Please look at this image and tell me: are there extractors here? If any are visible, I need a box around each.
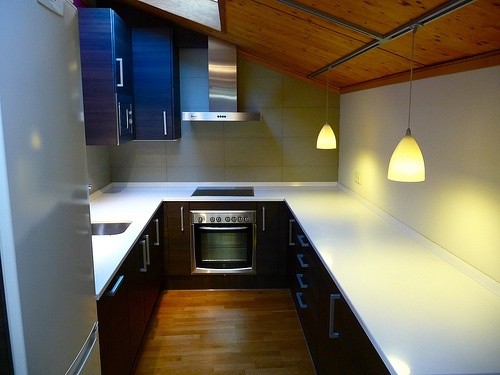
[182,35,262,121]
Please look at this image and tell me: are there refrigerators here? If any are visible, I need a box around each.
[1,0,101,375]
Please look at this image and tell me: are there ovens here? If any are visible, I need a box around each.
[189,210,257,276]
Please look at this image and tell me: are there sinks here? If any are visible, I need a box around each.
[91,224,130,236]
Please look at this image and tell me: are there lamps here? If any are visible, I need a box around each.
[317,67,338,149]
[388,25,428,183]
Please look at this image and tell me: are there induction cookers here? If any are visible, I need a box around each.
[191,187,255,196]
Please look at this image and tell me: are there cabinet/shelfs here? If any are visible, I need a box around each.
[257,202,291,278]
[131,28,184,141]
[280,215,321,363]
[126,230,162,348]
[164,202,191,278]
[97,267,134,375]
[317,269,386,375]
[79,7,134,147]
[148,210,169,293]
